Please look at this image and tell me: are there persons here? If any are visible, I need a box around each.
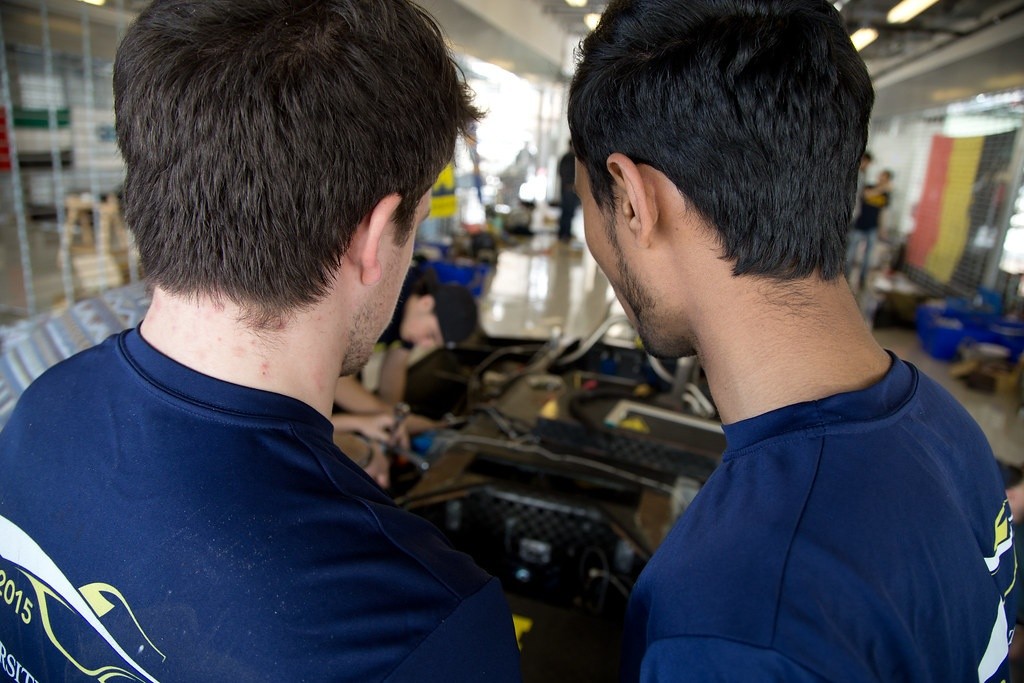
[1,1,524,683]
[845,153,895,289]
[559,141,579,239]
[570,1,1024,683]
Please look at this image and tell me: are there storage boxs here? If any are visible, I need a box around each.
[428,263,487,295]
[867,271,1024,363]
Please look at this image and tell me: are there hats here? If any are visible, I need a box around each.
[422,272,478,349]
